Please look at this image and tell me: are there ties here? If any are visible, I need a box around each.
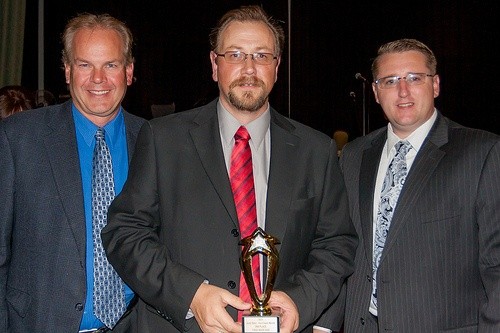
[371,140,413,308]
[230,126,262,322]
[91,127,128,330]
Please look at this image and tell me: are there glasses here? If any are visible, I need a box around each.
[217,51,277,64]
[375,73,433,88]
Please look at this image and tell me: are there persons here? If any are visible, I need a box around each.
[0,14,148,333]
[0,84,32,118]
[312,38,500,333]
[100,6,360,333]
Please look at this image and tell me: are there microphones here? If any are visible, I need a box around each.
[355,72,367,82]
